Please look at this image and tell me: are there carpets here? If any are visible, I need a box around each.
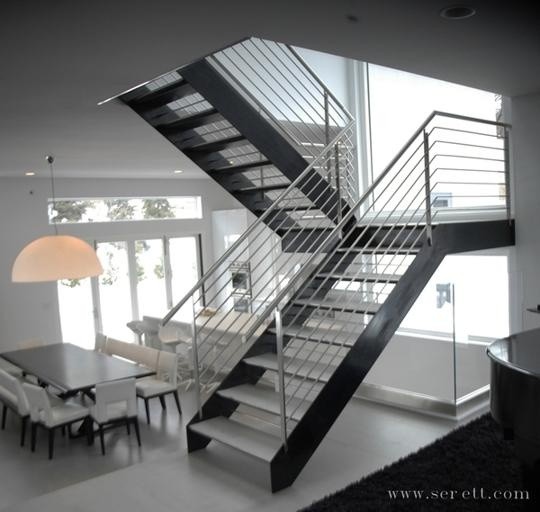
[296,410,539,512]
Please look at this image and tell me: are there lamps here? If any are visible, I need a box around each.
[11,154,104,283]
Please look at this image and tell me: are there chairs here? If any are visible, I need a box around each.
[127,320,228,392]
[1,332,183,460]
